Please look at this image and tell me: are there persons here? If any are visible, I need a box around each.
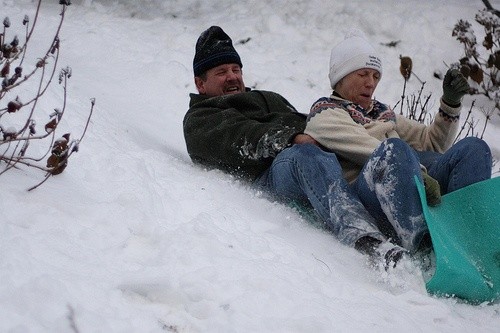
[303,37,493,206]
[182,25,410,273]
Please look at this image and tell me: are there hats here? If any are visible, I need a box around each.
[328,36,383,90]
[193,26,242,77]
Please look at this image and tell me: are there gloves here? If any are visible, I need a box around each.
[421,169,442,206]
[442,68,470,108]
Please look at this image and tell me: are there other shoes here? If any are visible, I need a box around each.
[375,238,412,274]
[420,246,437,284]
